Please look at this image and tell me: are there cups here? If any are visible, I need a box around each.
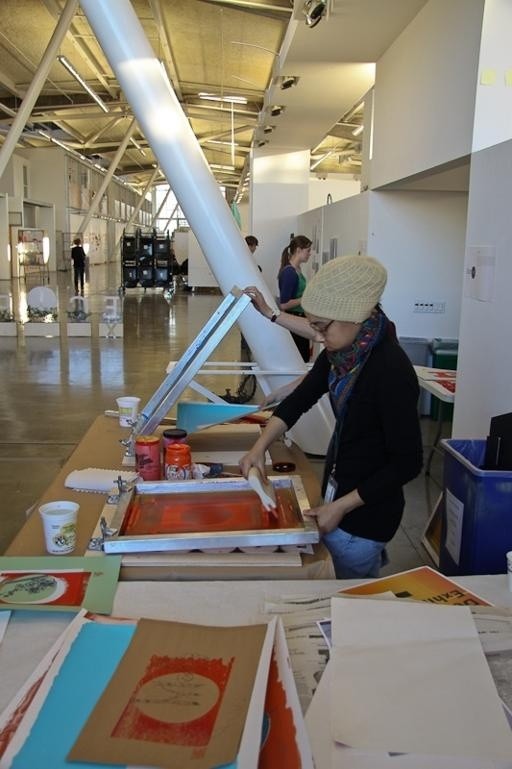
[114,395,142,427]
[37,499,82,556]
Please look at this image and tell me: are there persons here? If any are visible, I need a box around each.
[241,234,316,362]
[71,238,86,296]
[239,254,424,579]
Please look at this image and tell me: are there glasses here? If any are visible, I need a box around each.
[308,320,334,332]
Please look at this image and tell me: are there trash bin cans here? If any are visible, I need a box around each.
[439,438,512,576]
[399,337,458,422]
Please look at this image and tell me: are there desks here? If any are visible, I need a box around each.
[5,412,334,582]
[418,376,455,477]
[5,573,511,717]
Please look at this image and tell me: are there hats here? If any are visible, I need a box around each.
[300,256,388,323]
